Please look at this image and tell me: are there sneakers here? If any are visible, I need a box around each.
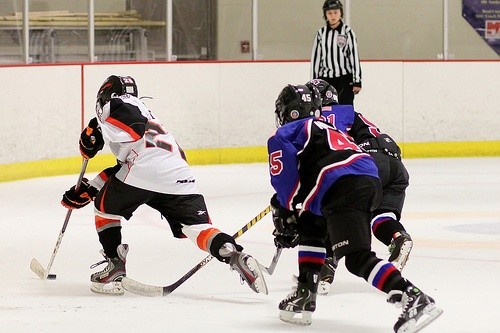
[388,230,413,273]
[278,272,319,326]
[219,242,269,295]
[386,278,443,333]
[317,253,338,294]
[90,244,129,296]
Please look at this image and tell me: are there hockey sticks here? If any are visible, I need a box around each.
[257,247,282,275]
[30,136,95,280]
[121,205,272,297]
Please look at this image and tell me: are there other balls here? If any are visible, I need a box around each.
[47,274,56,279]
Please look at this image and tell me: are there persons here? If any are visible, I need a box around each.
[60,74,268,296]
[310,0,362,105]
[260,83,446,333]
[290,78,413,291]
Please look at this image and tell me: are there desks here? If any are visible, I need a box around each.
[1,26,143,61]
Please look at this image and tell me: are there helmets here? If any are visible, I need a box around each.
[323,0,343,19]
[97,75,138,103]
[304,79,338,105]
[275,84,321,121]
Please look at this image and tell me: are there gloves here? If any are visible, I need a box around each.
[79,118,104,160]
[61,178,100,210]
[272,229,299,248]
[270,193,298,236]
